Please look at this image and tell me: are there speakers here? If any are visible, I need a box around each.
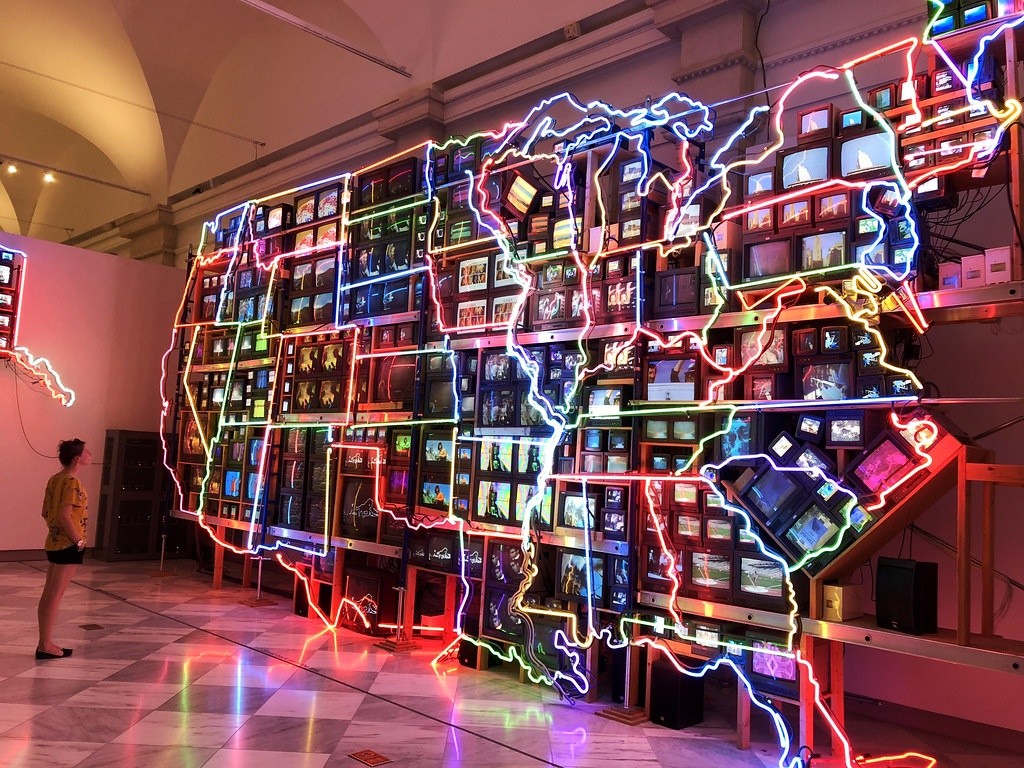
[295,577,309,618]
[650,659,704,731]
[459,639,503,669]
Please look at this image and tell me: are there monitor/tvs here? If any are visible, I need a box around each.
[175,0,1024,702]
[0,249,15,356]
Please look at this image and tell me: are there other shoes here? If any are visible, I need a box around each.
[35,647,73,659]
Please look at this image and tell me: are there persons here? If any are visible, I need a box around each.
[0,252,15,347]
[298,259,757,594]
[34,437,93,660]
